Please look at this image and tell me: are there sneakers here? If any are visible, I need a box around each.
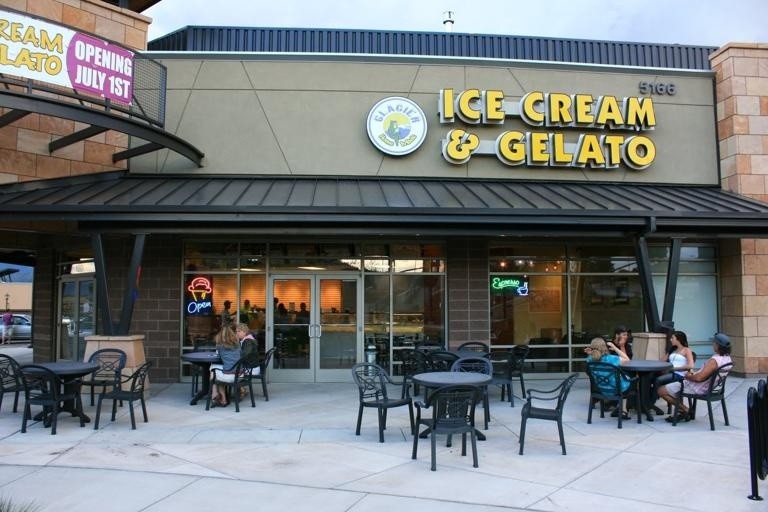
[611,408,631,419]
[665,408,690,422]
[211,394,228,408]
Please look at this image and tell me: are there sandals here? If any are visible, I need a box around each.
[240,388,248,400]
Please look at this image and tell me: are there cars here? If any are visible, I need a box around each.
[0,311,31,341]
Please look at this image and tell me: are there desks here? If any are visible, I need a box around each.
[616,360,673,421]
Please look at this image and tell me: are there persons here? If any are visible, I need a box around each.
[219,297,310,346]
[495,319,513,345]
[656,333,733,423]
[606,325,637,408]
[649,331,694,408]
[1,309,13,345]
[234,324,260,398]
[583,337,632,420]
[209,326,244,407]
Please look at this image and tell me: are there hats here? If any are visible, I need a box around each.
[709,333,731,348]
[224,301,232,305]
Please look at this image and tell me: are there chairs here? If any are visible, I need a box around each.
[95,360,154,429]
[519,373,578,455]
[0,353,32,419]
[663,351,697,413]
[586,361,641,427]
[350,333,530,471]
[77,348,127,413]
[17,365,85,435]
[673,362,734,431]
[190,331,289,412]
